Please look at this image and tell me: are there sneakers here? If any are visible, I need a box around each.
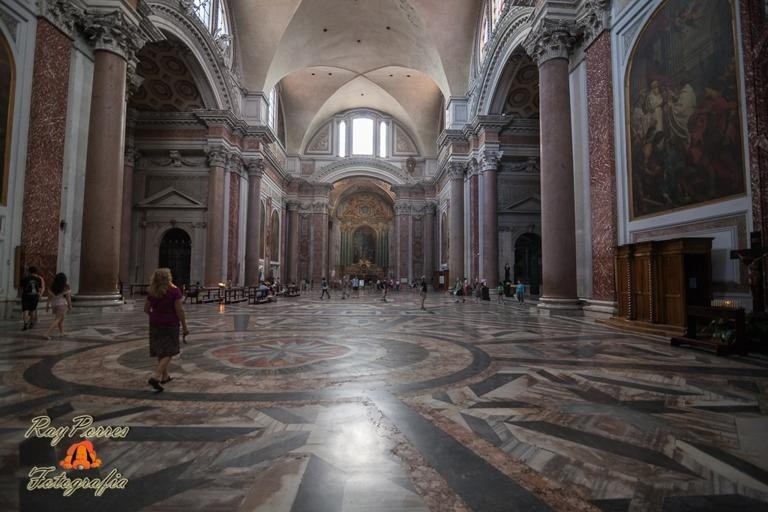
[23,322,33,330]
[42,333,51,340]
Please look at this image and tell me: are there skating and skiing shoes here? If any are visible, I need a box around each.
[60,332,68,337]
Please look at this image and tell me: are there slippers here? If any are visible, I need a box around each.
[148,378,164,392]
[160,375,175,384]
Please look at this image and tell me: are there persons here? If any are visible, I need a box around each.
[319,273,401,302]
[419,275,428,312]
[250,278,313,300]
[497,282,507,305]
[183,279,201,304]
[509,278,526,305]
[19,265,75,341]
[447,275,486,304]
[142,268,191,396]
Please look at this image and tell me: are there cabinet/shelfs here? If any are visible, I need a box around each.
[131,281,304,306]
